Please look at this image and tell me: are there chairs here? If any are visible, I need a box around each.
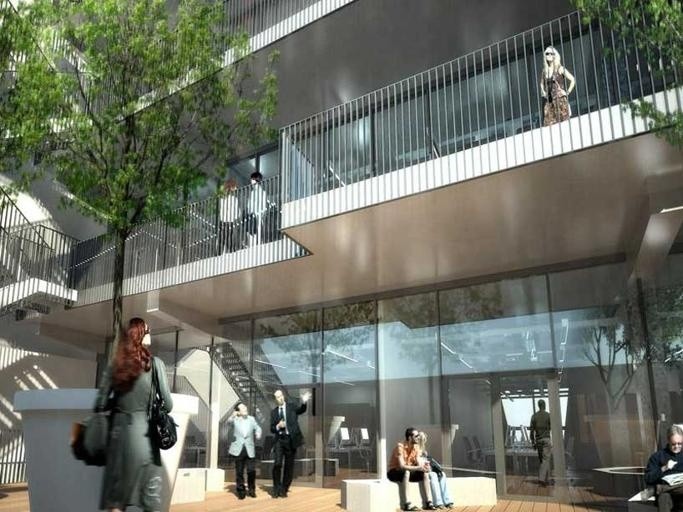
[304,432,376,472]
[463,436,574,474]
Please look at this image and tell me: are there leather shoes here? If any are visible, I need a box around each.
[249,492,256,497]
[239,493,246,499]
[273,490,287,498]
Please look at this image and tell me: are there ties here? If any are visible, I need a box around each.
[280,408,285,436]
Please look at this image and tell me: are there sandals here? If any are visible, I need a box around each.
[425,500,453,510]
[403,502,422,512]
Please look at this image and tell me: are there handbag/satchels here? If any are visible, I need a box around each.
[152,404,179,450]
[429,458,443,477]
[71,414,108,466]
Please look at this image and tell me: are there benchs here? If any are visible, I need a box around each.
[259,456,340,480]
[341,477,497,511]
[171,467,226,505]
[627,488,659,512]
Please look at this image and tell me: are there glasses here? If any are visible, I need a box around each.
[143,326,151,334]
[545,53,556,55]
[670,443,683,445]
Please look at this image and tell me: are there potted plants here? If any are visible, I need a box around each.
[0,0,281,512]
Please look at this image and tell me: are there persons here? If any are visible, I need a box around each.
[96,317,172,512]
[270,389,312,498]
[226,403,262,500]
[645,425,683,512]
[530,399,550,486]
[541,47,575,126]
[217,180,241,256]
[244,171,267,248]
[388,427,454,511]
